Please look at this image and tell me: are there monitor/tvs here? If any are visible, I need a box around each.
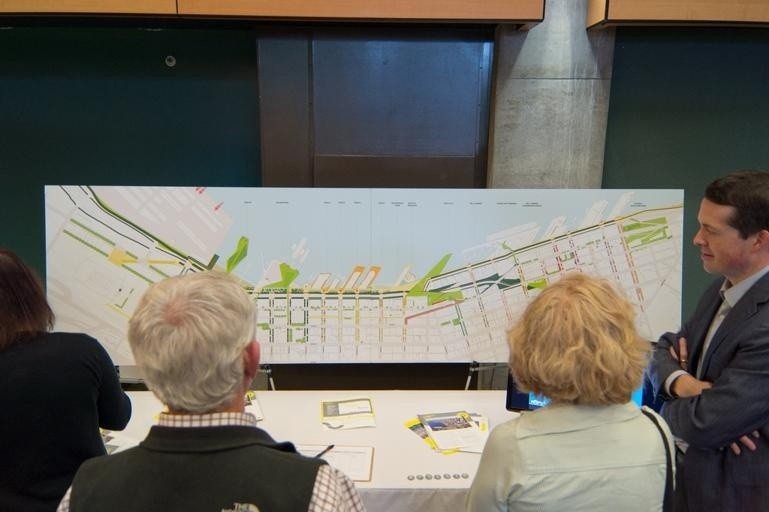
[506,341,660,412]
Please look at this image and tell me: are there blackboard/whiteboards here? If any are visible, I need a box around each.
[601,24,769,338]
[1,11,500,306]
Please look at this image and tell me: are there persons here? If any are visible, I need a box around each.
[645,168,768,511]
[0,248,132,512]
[56,268,365,511]
[466,272,676,512]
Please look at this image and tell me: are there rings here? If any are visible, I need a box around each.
[679,360,688,363]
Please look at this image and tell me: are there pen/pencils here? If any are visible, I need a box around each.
[312,443,335,459]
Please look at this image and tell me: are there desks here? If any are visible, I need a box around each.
[58,390,521,511]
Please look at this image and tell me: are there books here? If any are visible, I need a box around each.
[321,399,376,430]
[242,390,262,421]
[290,444,375,482]
[401,409,489,455]
